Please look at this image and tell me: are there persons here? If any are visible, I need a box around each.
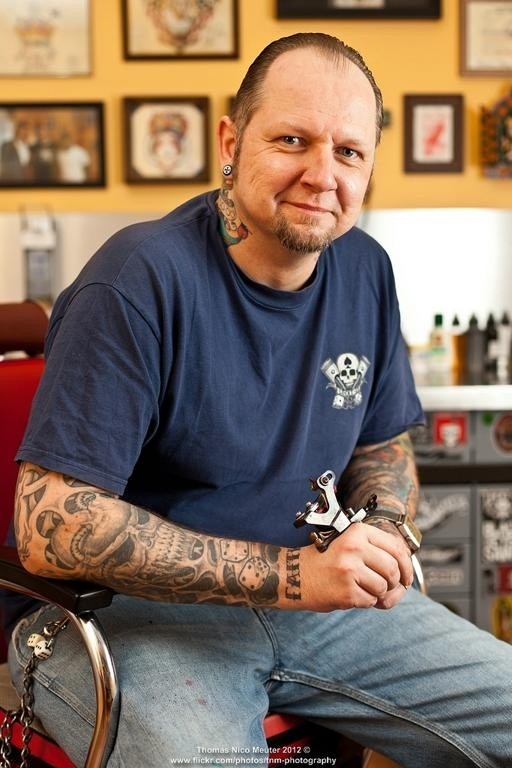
[11,30,511,768]
[47,129,94,182]
[2,122,39,182]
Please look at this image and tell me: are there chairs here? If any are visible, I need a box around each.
[0,297,324,766]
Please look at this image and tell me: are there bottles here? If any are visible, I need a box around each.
[428,310,512,386]
[19,197,66,318]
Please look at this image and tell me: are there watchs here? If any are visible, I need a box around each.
[363,506,424,554]
